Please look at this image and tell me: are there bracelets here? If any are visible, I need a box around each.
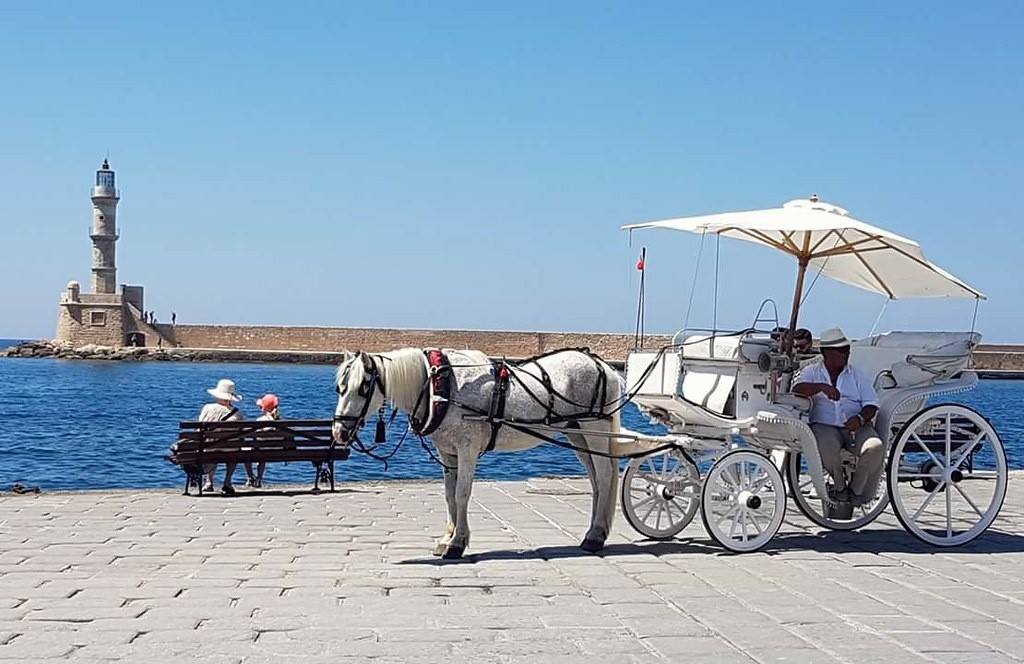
[856,413,864,427]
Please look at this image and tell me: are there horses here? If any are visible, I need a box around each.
[332,348,623,560]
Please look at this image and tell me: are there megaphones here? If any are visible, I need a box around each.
[757,352,791,374]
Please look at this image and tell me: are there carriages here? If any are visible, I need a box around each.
[333,194,1010,560]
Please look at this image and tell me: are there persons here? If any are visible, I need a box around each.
[242,394,280,487]
[776,329,820,393]
[792,328,884,507]
[196,379,243,494]
[771,327,795,358]
[132,311,176,351]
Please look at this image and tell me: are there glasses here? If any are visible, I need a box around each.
[827,345,850,354]
[794,341,809,350]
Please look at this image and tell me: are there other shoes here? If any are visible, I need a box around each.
[222,483,235,494]
[202,482,214,492]
[254,478,261,487]
[829,481,848,501]
[246,479,253,486]
[848,486,863,507]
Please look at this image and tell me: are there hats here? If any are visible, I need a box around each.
[257,394,279,412]
[207,379,242,402]
[812,328,852,347]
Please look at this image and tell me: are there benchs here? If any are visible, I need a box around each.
[164,420,351,497]
[795,330,983,423]
[671,298,779,374]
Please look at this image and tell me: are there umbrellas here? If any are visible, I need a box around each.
[620,192,986,332]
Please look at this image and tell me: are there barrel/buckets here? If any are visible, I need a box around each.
[821,475,854,519]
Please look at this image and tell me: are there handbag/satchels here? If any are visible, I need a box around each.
[284,435,296,450]
[170,438,197,455]
